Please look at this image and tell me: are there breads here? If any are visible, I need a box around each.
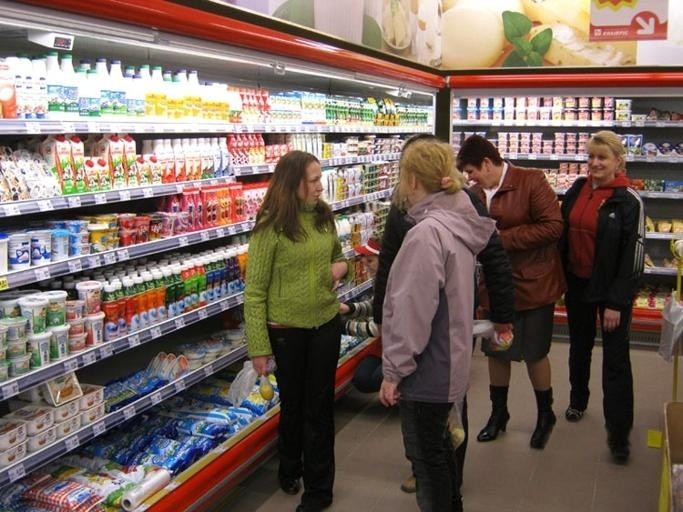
[441,0,638,70]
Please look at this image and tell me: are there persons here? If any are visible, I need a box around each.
[455,134,569,449]
[371,135,498,511]
[373,135,515,493]
[242,149,356,512]
[560,130,645,467]
[339,236,383,393]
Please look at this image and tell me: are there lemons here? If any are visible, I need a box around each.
[260,379,274,400]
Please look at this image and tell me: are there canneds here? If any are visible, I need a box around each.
[564,96,615,121]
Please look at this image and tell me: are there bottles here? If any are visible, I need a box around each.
[0,51,270,122]
[37,242,251,344]
[154,180,271,236]
[140,132,266,185]
[334,213,352,251]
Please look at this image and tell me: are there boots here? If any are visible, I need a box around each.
[478,385,509,441]
[531,387,556,448]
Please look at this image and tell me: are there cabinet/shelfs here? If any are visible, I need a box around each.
[449,64,683,355]
[0,0,449,511]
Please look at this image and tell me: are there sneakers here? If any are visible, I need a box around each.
[281,477,299,494]
[566,405,584,421]
[402,476,416,492]
